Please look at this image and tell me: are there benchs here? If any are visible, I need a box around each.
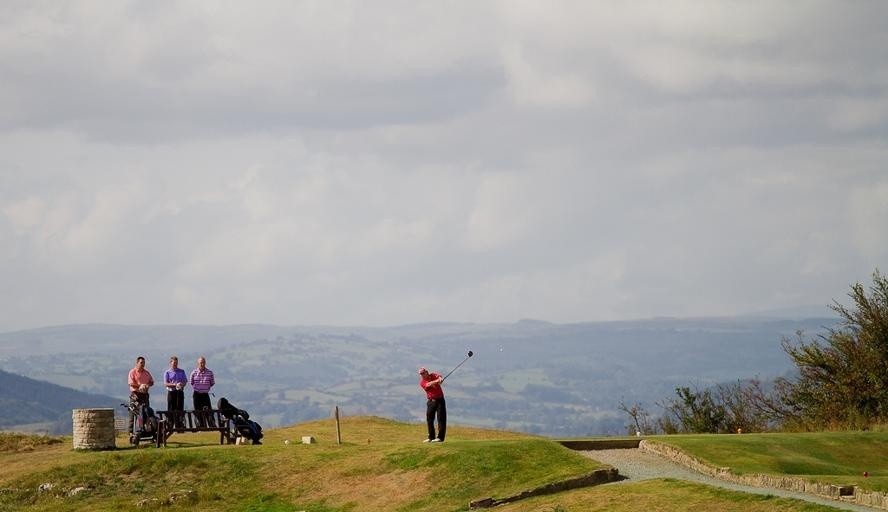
[155,409,233,448]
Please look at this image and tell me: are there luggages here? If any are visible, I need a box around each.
[217,397,264,444]
[129,401,165,447]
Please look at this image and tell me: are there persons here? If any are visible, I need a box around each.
[163,357,188,433]
[417,366,448,443]
[126,356,156,437]
[190,355,218,428]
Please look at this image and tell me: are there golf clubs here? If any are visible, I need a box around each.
[443,351,473,379]
[120,402,139,415]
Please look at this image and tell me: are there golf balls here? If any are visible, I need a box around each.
[284,440,289,444]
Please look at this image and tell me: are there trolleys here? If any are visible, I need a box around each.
[125,408,163,447]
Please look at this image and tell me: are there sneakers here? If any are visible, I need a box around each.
[423,439,442,443]
